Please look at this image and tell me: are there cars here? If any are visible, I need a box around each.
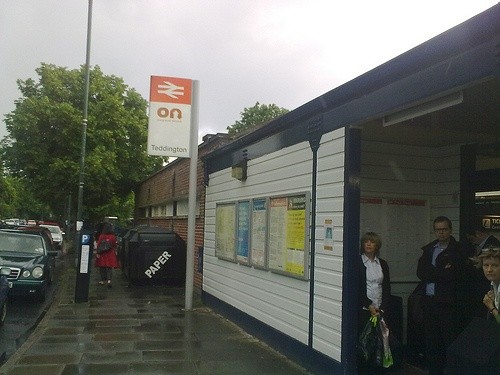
[0,218,66,324]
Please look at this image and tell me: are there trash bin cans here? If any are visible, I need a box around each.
[121,226,187,285]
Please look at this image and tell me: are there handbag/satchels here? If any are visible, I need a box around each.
[98,243,110,253]
[360,317,393,368]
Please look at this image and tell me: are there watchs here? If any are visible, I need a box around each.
[489,307,497,314]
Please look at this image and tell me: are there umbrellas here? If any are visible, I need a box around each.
[444,317,500,375]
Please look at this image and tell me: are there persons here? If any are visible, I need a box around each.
[358,231,391,375]
[469,246,499,324]
[95,223,119,289]
[417,215,468,364]
[462,221,500,300]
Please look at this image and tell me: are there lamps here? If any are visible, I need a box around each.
[232,159,247,181]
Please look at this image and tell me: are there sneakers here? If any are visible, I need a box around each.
[108,280,110,283]
[99,281,103,284]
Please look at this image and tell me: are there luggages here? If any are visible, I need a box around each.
[386,294,402,367]
[408,280,428,368]
